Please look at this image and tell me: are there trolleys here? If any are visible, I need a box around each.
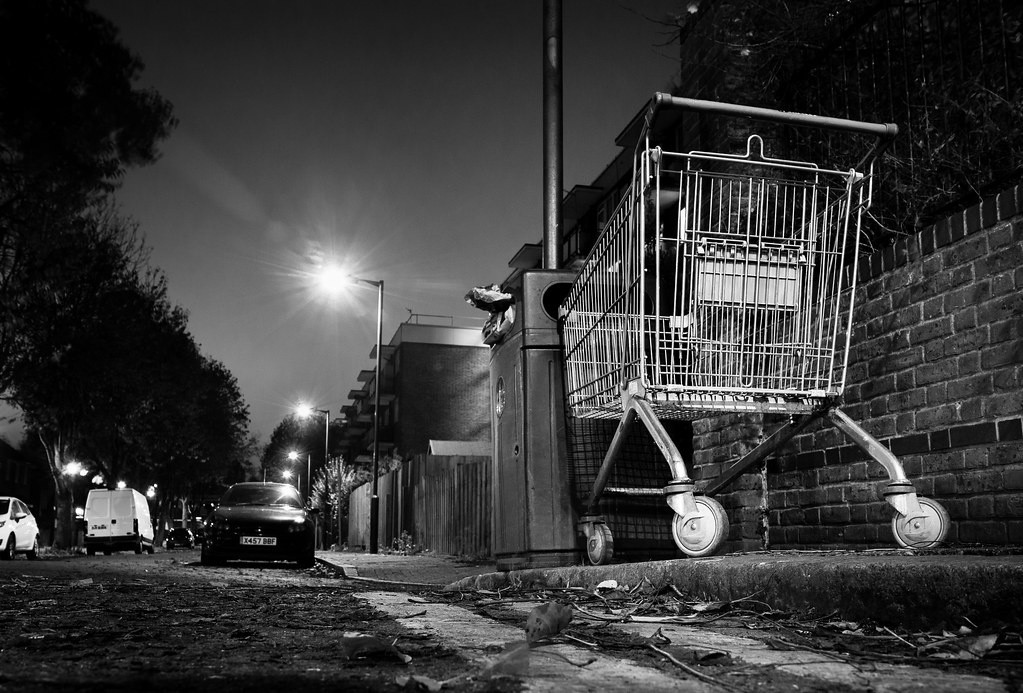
[558,83,953,563]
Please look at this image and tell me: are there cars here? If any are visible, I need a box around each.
[166,527,195,550]
[0,496,42,560]
[201,482,317,569]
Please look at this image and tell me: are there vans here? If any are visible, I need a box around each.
[81,485,156,554]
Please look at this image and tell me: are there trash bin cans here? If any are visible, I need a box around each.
[464,267,696,570]
